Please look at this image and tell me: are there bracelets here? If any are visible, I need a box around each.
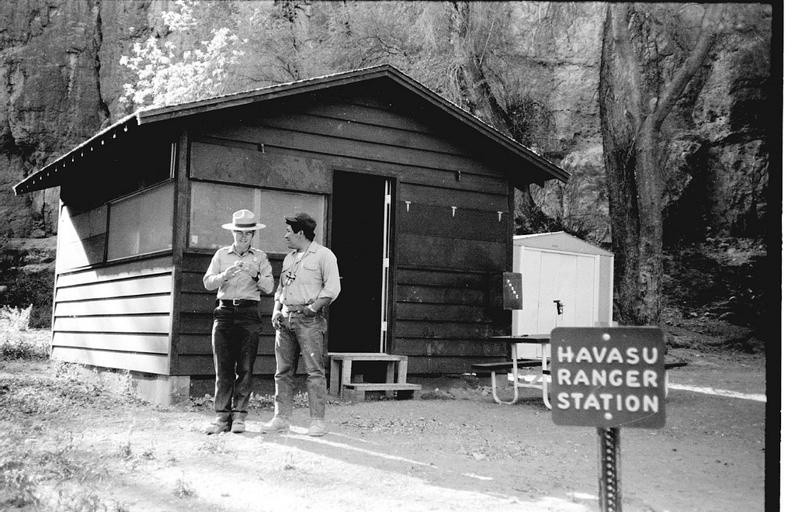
[307,304,317,314]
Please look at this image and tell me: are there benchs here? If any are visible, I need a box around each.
[472,358,550,403]
[542,360,687,399]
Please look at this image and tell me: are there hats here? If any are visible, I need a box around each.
[221,210,266,231]
[285,212,317,232]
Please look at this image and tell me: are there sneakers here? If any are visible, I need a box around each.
[204,419,245,434]
[309,419,327,436]
[260,416,291,433]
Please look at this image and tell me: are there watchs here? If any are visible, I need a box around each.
[251,272,262,282]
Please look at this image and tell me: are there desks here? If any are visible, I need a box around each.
[491,335,554,410]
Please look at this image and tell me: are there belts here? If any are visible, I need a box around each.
[219,299,257,307]
[282,305,304,312]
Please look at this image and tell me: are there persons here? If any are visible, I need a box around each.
[258,211,342,437]
[201,210,276,434]
